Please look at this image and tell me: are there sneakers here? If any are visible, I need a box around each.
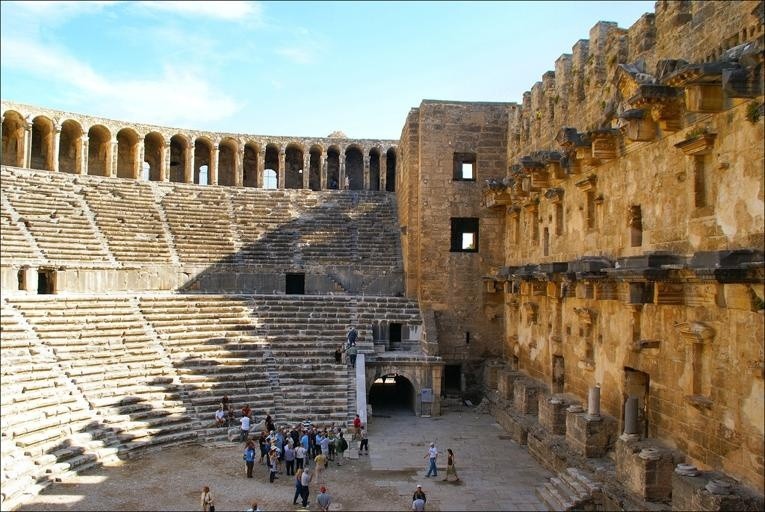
[424,474,438,478]
[292,501,310,509]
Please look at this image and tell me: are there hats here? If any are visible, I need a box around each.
[417,484,422,488]
[319,486,327,492]
[429,442,435,446]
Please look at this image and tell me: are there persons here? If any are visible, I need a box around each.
[442,449,462,482]
[247,503,261,512]
[215,392,235,428]
[423,442,439,477]
[201,486,214,512]
[328,175,349,190]
[293,468,313,507]
[316,487,331,512]
[334,328,357,368]
[240,403,368,483]
[411,484,426,512]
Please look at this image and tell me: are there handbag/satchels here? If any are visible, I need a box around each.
[210,506,215,512]
